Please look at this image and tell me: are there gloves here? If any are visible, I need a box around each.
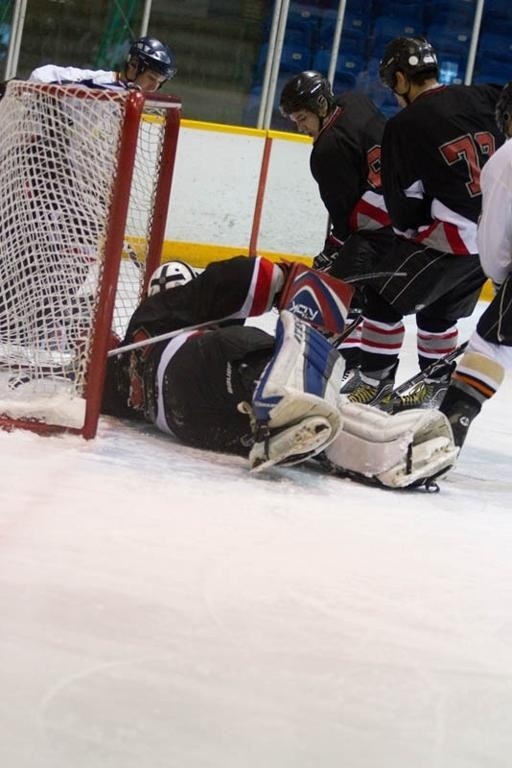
[311,237,341,271]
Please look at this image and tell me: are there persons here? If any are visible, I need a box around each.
[437,78,512,481]
[346,32,505,414]
[1,36,179,355]
[84,257,456,490]
[277,70,390,397]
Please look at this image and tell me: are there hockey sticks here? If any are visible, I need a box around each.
[8,271,409,390]
[367,339,470,415]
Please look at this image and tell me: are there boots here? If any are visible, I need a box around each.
[344,361,397,407]
[338,364,360,394]
[393,361,458,414]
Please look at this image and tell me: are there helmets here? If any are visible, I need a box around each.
[146,260,196,296]
[278,70,331,119]
[126,37,178,84]
[377,35,439,86]
